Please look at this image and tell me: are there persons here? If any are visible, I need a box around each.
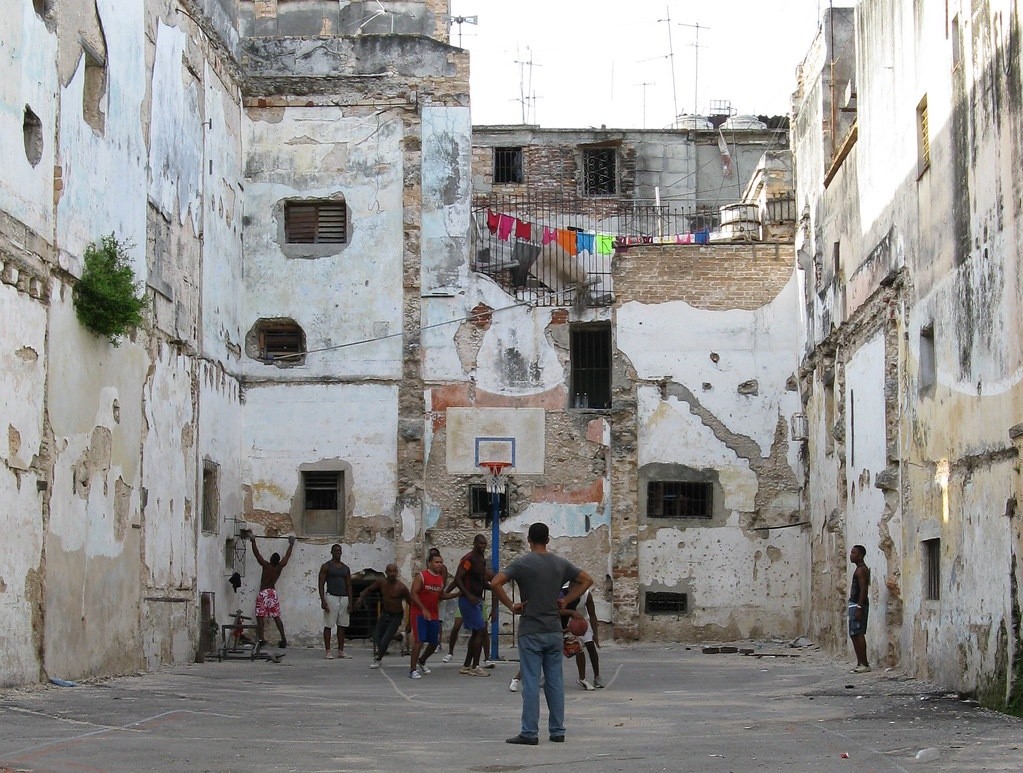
[491,522,594,746]
[246,533,295,648]
[508,579,581,692]
[401,548,447,656]
[318,543,354,660]
[354,562,411,669]
[561,580,605,688]
[408,552,463,682]
[454,533,490,677]
[846,544,872,674]
[444,577,494,668]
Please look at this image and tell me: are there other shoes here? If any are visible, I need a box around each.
[254,639,266,648]
[550,735,565,742]
[505,735,538,745]
[279,640,286,647]
[850,665,858,674]
[854,663,871,673]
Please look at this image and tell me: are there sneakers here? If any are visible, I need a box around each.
[337,650,353,658]
[369,659,382,668]
[409,670,422,679]
[416,659,430,673]
[484,660,496,668]
[593,677,605,687]
[460,665,471,673]
[576,677,596,690]
[469,665,490,677]
[510,678,519,692]
[443,654,453,663]
[325,650,333,659]
[399,631,408,648]
[540,677,546,687]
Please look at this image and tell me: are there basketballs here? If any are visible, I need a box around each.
[570,618,588,636]
